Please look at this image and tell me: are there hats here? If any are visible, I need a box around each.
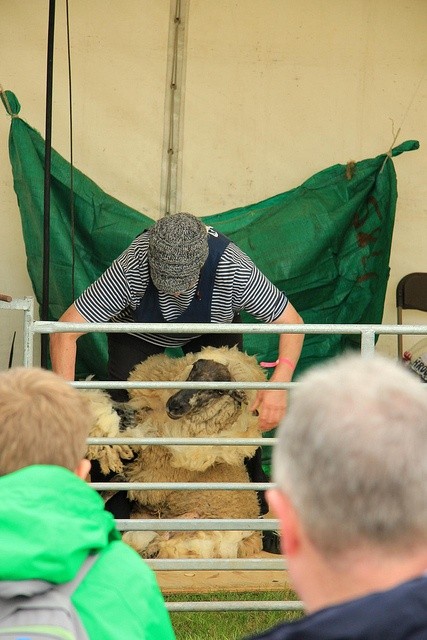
[148,212,209,292]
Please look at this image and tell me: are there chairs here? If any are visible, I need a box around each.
[395,272,427,383]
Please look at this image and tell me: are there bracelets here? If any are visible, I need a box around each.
[259,354,298,369]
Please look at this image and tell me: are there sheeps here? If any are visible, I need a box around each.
[100,345,271,559]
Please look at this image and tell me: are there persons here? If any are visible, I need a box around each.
[1,367,175,640]
[248,350,425,640]
[49,212,305,483]
